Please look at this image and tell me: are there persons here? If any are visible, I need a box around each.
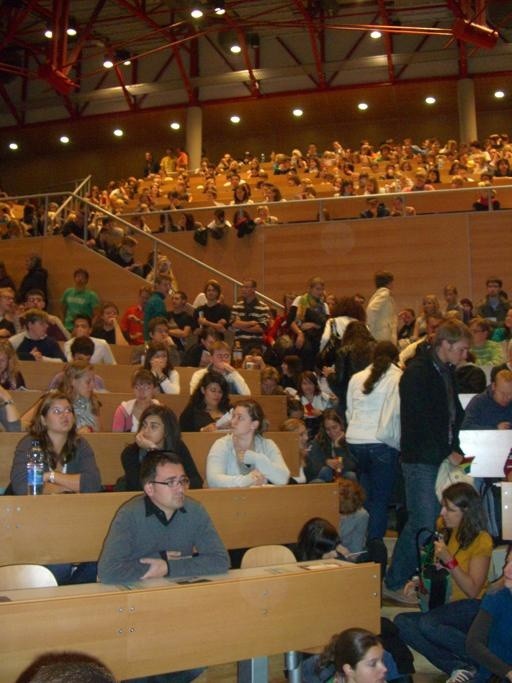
[1,185,159,277]
[109,133,512,231]
[1,253,511,683]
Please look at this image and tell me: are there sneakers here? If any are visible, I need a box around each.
[447,667,479,683]
[381,580,420,604]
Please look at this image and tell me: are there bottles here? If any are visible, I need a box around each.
[233,340,246,369]
[260,153,265,162]
[199,311,204,327]
[26,440,43,495]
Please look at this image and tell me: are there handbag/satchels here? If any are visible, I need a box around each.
[316,336,342,374]
[416,537,450,612]
[435,459,473,502]
[376,379,400,453]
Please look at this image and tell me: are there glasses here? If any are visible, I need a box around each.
[149,478,190,487]
